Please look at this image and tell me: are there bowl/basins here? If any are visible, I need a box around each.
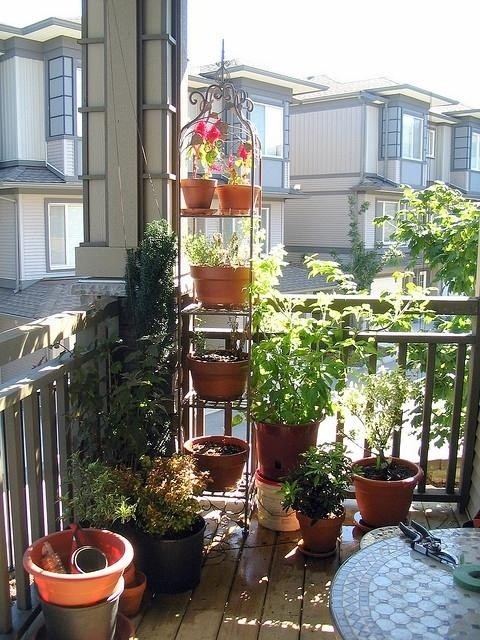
[23,529,136,607]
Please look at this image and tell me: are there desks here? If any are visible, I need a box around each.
[329,526,480,640]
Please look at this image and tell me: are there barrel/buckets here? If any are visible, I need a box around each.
[253,479,301,533]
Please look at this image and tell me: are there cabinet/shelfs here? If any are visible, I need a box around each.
[177,61,262,537]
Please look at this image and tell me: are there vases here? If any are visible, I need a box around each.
[116,613,139,640]
[216,184,262,214]
[180,177,217,209]
[192,267,253,308]
[118,571,147,616]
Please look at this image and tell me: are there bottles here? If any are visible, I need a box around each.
[41,541,67,575]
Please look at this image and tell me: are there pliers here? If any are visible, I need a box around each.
[399,520,458,568]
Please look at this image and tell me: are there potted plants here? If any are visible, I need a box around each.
[334,365,425,525]
[113,454,214,591]
[186,315,253,401]
[231,253,440,481]
[273,442,364,552]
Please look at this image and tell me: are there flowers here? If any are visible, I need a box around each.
[181,230,249,266]
[182,117,222,179]
[224,143,251,185]
